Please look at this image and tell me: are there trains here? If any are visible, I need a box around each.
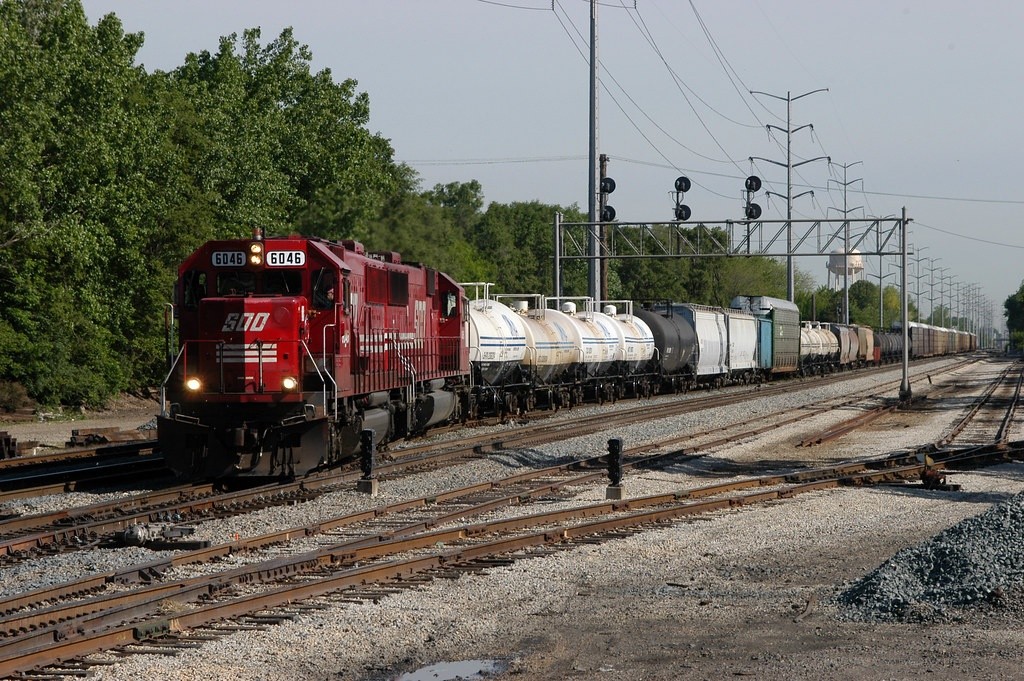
[155,223,982,499]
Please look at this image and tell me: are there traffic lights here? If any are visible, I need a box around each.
[606,437,624,482]
[359,428,377,476]
